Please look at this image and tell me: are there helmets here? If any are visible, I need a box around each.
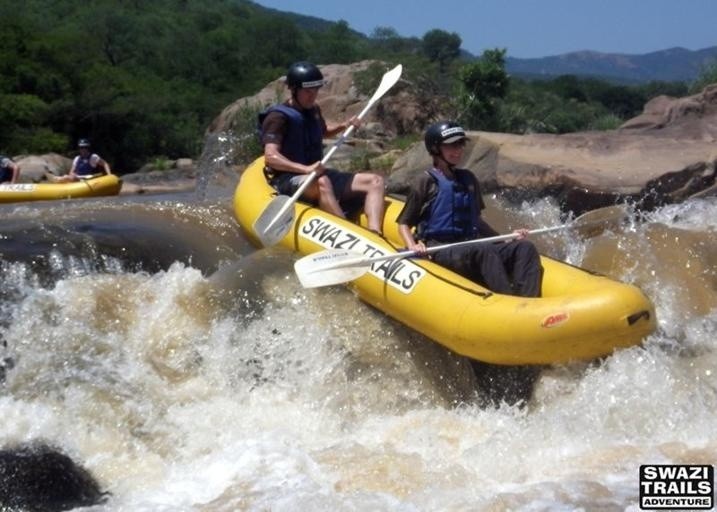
[78,139,90,147]
[425,121,471,156]
[288,63,323,89]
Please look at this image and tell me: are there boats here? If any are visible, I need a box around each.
[0,173,124,204]
[231,154,659,367]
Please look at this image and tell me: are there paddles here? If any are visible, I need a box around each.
[44,171,103,184]
[253,62,404,245]
[293,207,621,289]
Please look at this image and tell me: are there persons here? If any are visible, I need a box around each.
[256,60,386,234]
[396,122,543,297]
[70,139,111,178]
[0,156,19,185]
